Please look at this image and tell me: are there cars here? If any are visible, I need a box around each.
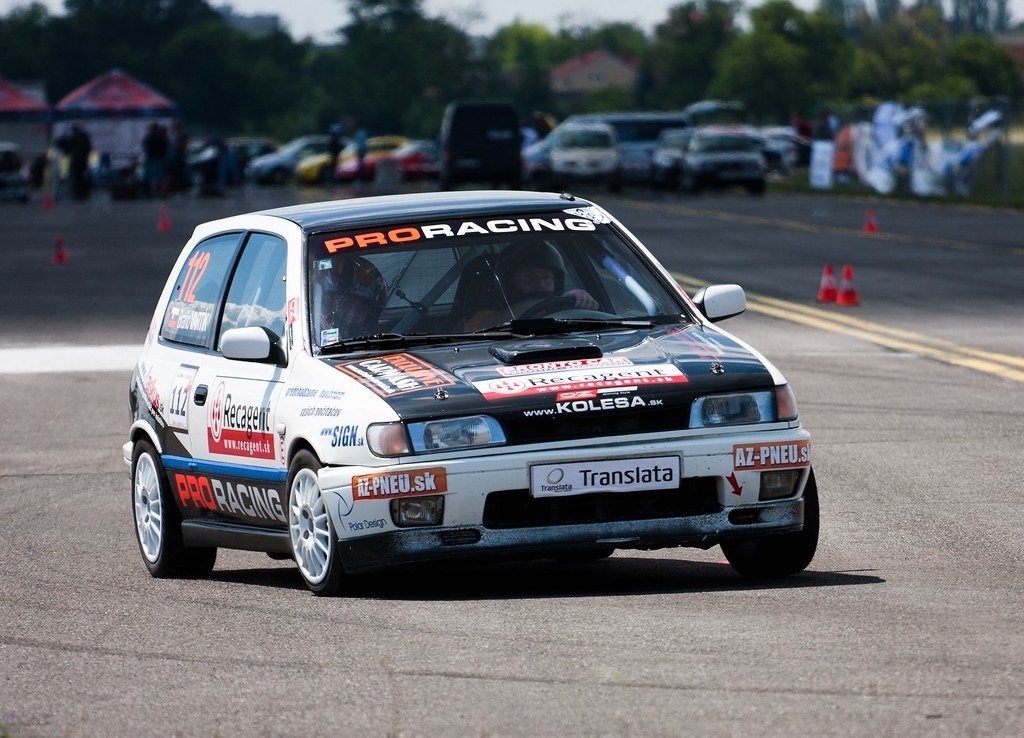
[182,99,801,198]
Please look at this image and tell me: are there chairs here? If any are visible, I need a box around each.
[446,253,497,333]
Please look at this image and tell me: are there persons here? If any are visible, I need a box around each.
[464,239,599,332]
[314,257,387,340]
[61,107,929,200]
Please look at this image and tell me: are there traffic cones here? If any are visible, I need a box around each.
[862,208,878,233]
[155,206,171,230]
[816,263,838,303]
[42,190,53,210]
[52,233,69,264]
[837,263,860,308]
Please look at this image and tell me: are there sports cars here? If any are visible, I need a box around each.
[119,188,821,597]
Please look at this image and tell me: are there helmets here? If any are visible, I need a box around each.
[498,239,566,303]
[314,255,387,339]
